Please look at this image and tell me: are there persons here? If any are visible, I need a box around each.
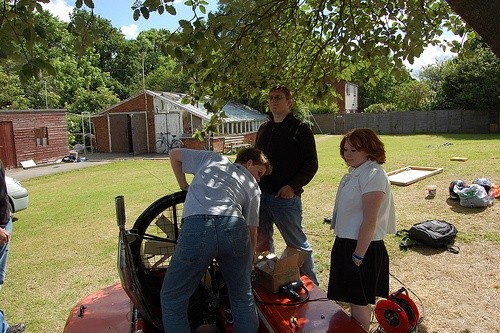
[0,157,25,333]
[253,86,321,287]
[160,146,272,333]
[327,127,396,333]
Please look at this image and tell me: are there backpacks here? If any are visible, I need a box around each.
[396,218,458,253]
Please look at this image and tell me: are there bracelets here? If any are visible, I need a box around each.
[352,252,364,261]
[180,184,189,190]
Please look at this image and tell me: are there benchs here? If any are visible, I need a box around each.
[5,175,29,212]
[223,135,252,155]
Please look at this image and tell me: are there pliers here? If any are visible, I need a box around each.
[290,313,298,329]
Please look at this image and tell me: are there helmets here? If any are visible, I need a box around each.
[448,180,464,200]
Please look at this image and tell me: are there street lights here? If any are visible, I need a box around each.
[142,51,149,152]
[40,80,48,109]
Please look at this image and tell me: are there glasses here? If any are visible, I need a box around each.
[268,95,283,100]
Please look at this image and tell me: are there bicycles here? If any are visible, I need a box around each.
[153,131,184,155]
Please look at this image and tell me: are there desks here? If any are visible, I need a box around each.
[253,273,370,333]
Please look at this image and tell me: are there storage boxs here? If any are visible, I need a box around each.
[254,247,308,294]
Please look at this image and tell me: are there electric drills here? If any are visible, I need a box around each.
[280,281,304,299]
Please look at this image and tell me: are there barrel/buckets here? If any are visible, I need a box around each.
[428,188,436,196]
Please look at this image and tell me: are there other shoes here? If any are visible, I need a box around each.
[6,323,25,333]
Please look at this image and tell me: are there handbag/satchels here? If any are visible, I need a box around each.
[453,183,489,208]
[473,178,492,193]
